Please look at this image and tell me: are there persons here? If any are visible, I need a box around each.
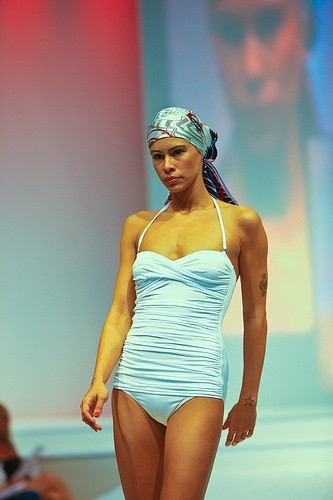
[0,403,74,500]
[198,0,333,416]
[78,107,269,500]
